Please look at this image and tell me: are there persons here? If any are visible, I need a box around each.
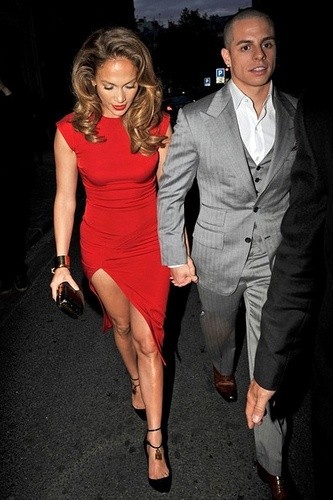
[155,10,298,500]
[245,79,333,500]
[49,26,195,492]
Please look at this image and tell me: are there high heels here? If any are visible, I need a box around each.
[143,427,171,493]
[130,377,147,421]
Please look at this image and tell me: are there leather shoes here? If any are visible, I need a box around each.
[213,365,238,402]
[267,473,289,500]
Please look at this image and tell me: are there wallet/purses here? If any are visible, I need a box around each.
[56,266,85,320]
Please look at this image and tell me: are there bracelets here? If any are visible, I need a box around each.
[54,254,71,268]
